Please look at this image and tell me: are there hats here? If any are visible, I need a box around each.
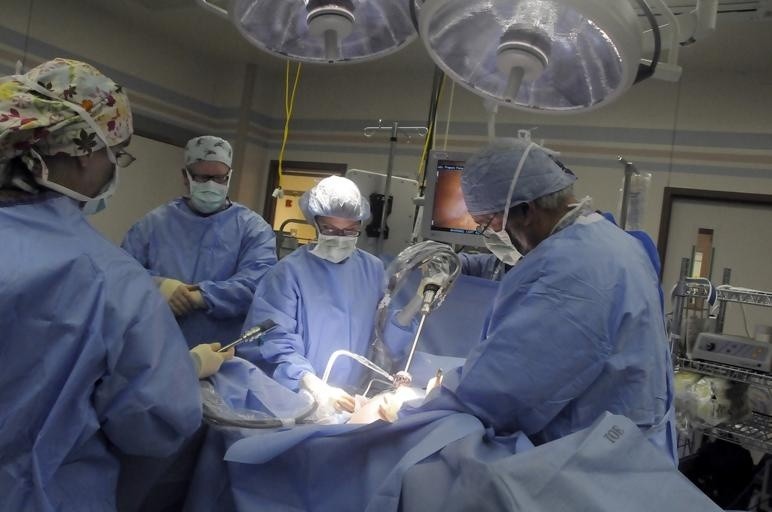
[0,57,135,165]
[183,135,234,169]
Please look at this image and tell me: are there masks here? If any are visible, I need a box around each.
[183,168,233,214]
[1,59,120,216]
[480,140,560,267]
[307,221,359,265]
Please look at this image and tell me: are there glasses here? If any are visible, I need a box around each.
[115,148,135,169]
[472,211,503,241]
[190,174,229,184]
[315,221,362,238]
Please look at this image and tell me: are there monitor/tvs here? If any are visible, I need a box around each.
[420,150,487,248]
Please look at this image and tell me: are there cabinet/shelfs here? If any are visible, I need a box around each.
[656,256,772,510]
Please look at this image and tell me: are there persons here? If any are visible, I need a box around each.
[237,173,393,412]
[379,136,681,473]
[457,154,664,316]
[0,56,206,512]
[120,134,281,512]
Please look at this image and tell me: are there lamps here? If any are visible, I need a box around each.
[197,0,726,115]
